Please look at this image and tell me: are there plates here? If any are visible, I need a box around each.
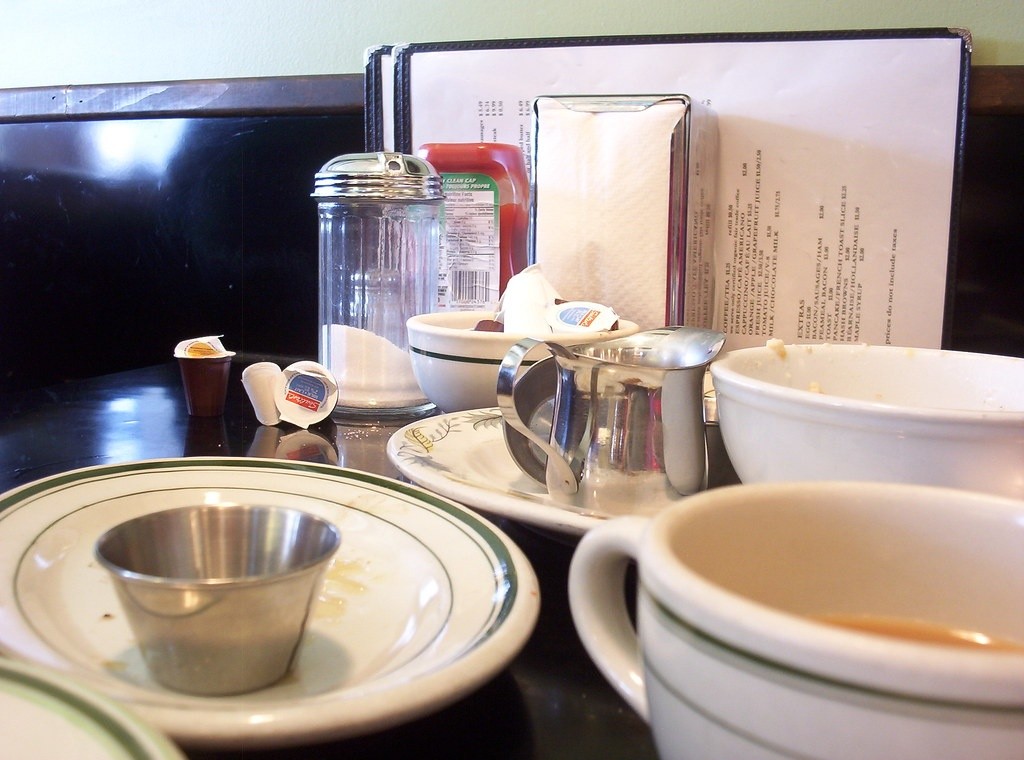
[0,457,540,747]
[0,657,188,760]
[386,406,648,535]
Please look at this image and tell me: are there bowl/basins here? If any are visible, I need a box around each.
[710,345,1024,502]
[407,311,640,413]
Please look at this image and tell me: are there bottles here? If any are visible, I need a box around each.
[310,151,443,415]
[418,143,530,309]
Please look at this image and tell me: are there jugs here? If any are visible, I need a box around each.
[498,326,726,519]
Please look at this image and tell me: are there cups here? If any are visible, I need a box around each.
[567,481,1024,760]
[96,503,339,695]
[173,351,236,416]
[702,390,742,490]
